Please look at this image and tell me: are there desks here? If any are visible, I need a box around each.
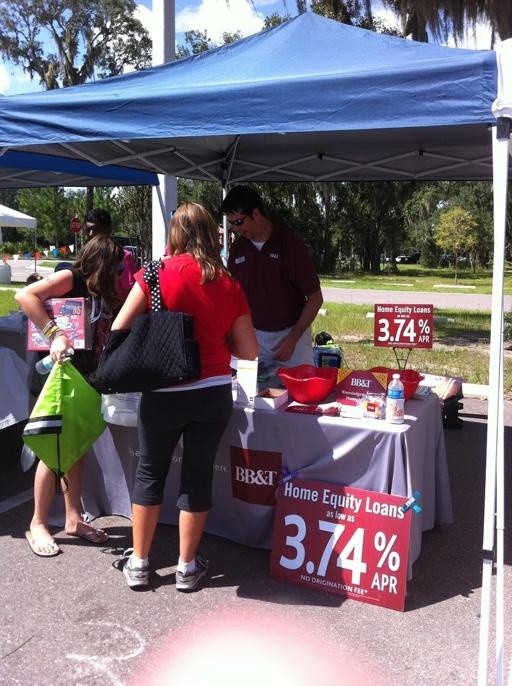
[82,384,452,594]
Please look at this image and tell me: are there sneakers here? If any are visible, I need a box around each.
[175,551,209,590]
[122,556,150,587]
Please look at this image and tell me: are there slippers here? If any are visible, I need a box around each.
[25,530,59,557]
[67,528,109,544]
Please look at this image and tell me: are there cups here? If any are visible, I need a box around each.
[386,398,405,424]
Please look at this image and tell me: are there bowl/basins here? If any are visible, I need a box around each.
[278,364,337,404]
[370,367,422,400]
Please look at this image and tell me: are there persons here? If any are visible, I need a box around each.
[111,199,260,592]
[82,208,138,317]
[221,186,325,384]
[216,224,230,268]
[15,232,125,556]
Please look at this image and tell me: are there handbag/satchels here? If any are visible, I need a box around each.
[22,356,108,476]
[94,311,201,394]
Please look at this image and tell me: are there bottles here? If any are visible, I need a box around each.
[34,347,74,375]
[388,373,404,398]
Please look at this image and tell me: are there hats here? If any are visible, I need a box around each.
[86,209,111,224]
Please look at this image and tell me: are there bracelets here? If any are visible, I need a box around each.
[42,320,64,342]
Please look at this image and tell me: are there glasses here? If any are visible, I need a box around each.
[227,214,247,226]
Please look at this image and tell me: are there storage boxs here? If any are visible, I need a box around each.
[26,299,96,351]
